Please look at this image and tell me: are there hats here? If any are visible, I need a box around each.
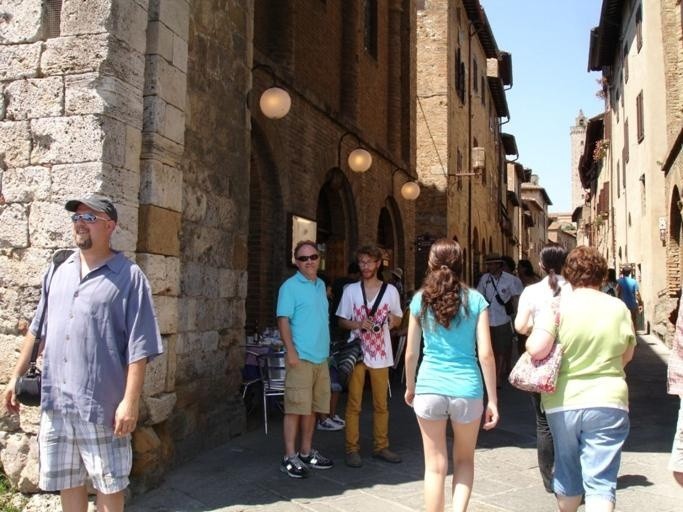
[64,193,117,225]
[483,255,504,264]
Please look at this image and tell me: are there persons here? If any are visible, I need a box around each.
[527,247,637,510]
[405,239,500,512]
[667,290,683,491]
[514,242,570,493]
[334,264,404,338]
[277,242,333,479]
[336,246,403,468]
[4,193,164,512]
[471,254,544,392]
[316,272,345,434]
[599,263,643,332]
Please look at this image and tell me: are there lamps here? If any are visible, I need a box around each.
[337,132,373,173]
[251,63,291,120]
[392,168,420,200]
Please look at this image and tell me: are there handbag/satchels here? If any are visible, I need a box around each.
[505,297,514,315]
[507,343,566,393]
[336,337,361,376]
[14,368,41,406]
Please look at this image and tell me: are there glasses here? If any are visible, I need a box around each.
[296,255,319,261]
[69,213,108,224]
[359,259,373,266]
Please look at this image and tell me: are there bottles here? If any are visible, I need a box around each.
[263,328,270,338]
[254,320,259,343]
[272,330,279,339]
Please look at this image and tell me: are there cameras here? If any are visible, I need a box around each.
[362,320,381,335]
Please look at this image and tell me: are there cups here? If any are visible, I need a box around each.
[247,337,253,343]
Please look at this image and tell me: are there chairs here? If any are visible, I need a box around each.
[240,349,283,414]
[256,351,288,440]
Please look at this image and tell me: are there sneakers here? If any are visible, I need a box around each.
[279,413,401,477]
